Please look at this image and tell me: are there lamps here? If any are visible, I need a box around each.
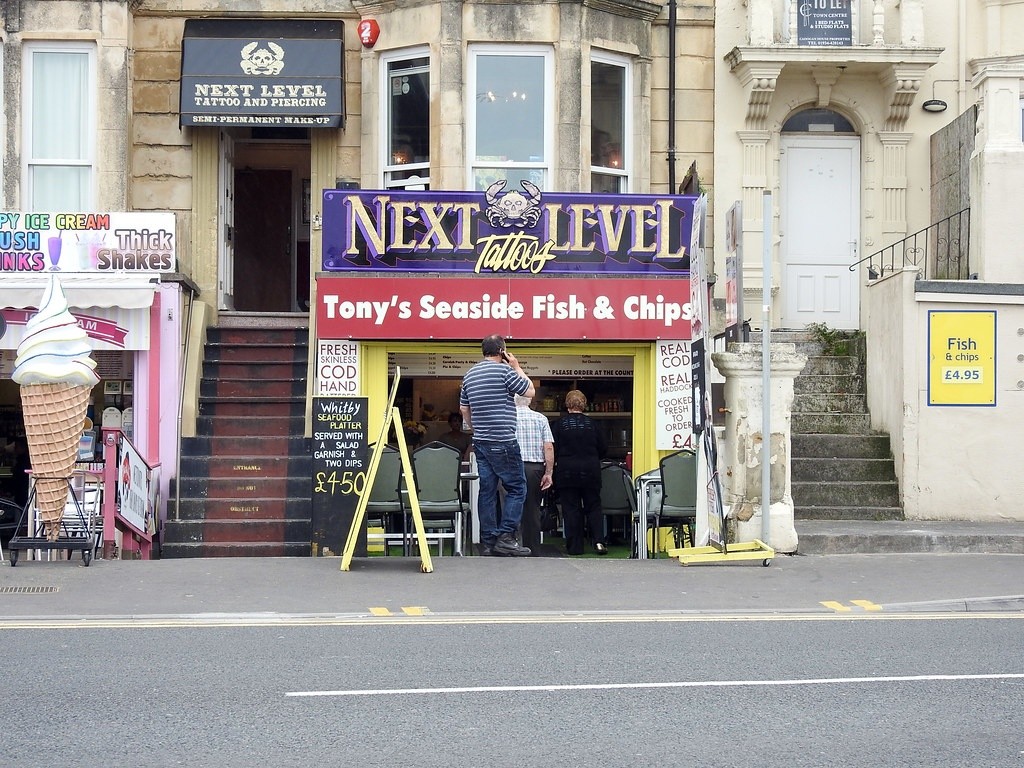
[922,100,947,111]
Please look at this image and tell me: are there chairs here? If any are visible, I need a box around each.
[366,441,470,557]
[598,449,698,560]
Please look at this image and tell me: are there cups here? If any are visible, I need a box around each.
[79,437,93,455]
[90,242,105,270]
[47,237,62,272]
[76,242,89,270]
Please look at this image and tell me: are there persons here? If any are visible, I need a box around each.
[439,411,470,457]
[513,393,555,556]
[551,390,608,555]
[459,334,537,556]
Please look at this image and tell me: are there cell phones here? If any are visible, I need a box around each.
[501,348,510,363]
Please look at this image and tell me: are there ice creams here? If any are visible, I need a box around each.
[12,273,100,541]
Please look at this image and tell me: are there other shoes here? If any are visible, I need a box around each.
[596,543,608,553]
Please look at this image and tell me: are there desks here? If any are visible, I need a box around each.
[636,474,663,560]
[400,472,479,557]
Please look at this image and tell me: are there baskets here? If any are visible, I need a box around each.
[543,396,557,411]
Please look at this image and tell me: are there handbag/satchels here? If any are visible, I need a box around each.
[540,487,560,531]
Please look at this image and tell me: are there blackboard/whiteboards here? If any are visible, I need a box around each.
[312,396,368,557]
[656,340,703,450]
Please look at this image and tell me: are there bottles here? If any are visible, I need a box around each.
[626,452,632,470]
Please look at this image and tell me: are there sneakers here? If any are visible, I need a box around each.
[480,542,494,555]
[493,533,531,555]
